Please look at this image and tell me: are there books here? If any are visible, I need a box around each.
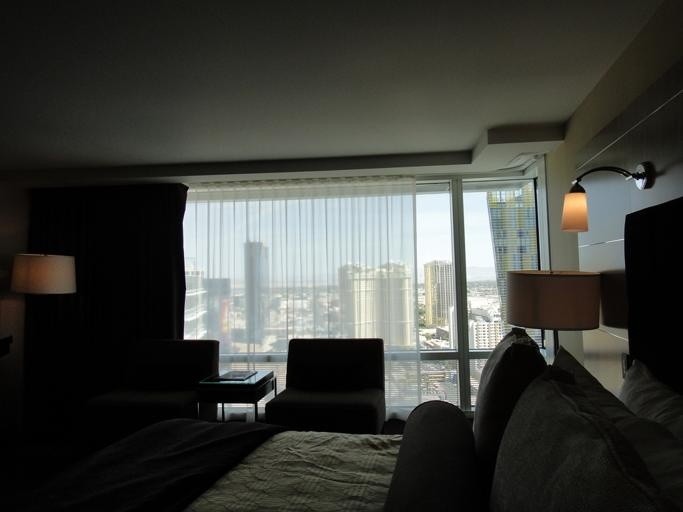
[215,371,257,380]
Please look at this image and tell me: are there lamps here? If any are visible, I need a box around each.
[9,251,77,294]
[560,161,656,232]
[507,270,601,356]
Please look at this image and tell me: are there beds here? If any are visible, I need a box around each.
[0,198,683,512]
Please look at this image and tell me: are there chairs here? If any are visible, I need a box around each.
[77,340,219,421]
[265,339,385,434]
